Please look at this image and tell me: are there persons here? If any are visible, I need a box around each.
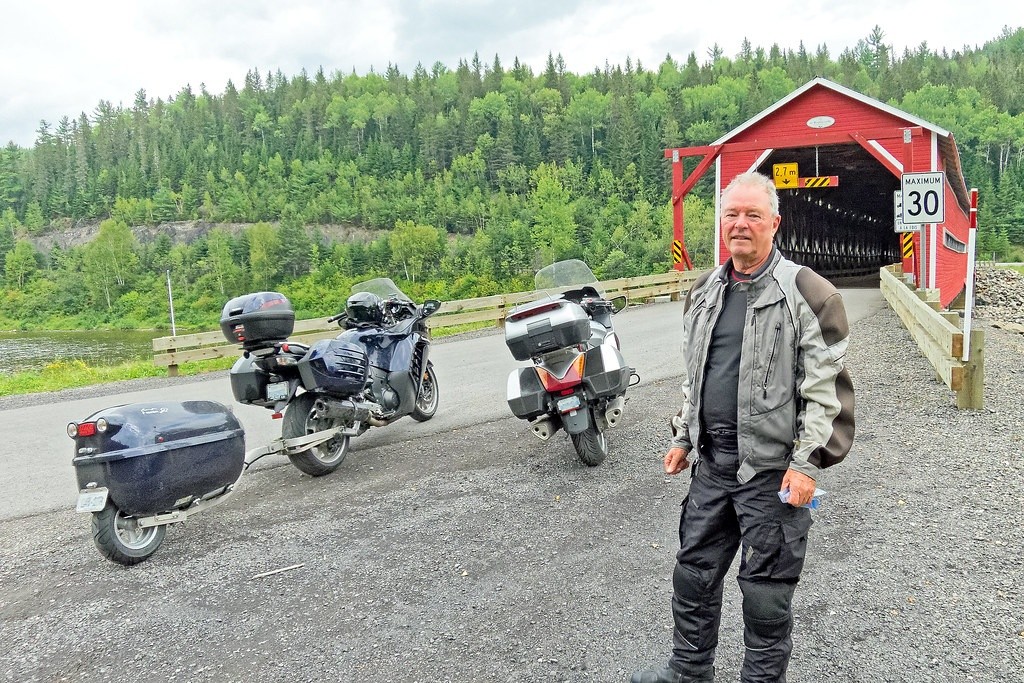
[629,172,855,683]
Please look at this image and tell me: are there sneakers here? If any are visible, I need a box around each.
[629,665,715,682]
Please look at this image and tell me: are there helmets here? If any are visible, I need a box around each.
[347,292,385,326]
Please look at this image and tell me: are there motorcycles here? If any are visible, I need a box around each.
[500,258,641,467]
[66,278,441,565]
